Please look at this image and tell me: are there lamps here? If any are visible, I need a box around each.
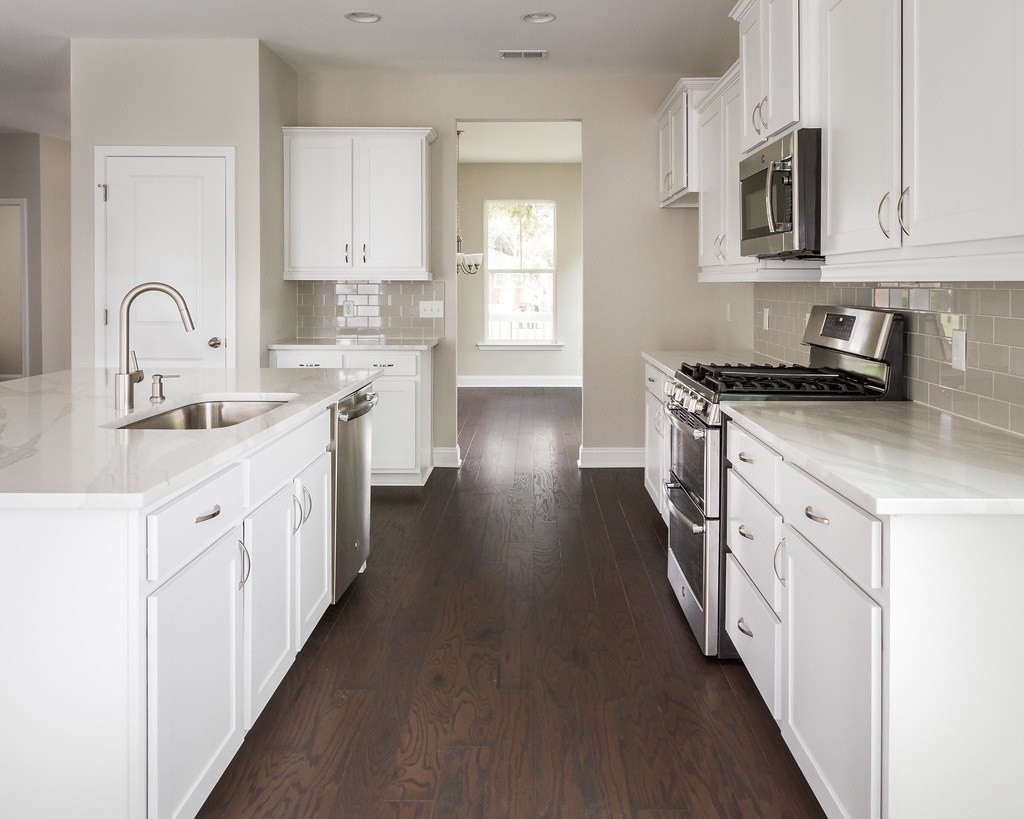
[457,130,484,275]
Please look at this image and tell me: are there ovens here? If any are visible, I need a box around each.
[664,389,720,656]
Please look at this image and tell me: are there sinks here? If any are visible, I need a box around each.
[100,391,300,429]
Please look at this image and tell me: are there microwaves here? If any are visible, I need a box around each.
[740,128,822,257]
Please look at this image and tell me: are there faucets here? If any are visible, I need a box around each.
[117,280,194,415]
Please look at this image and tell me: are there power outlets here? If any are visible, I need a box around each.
[343,302,354,318]
[952,329,965,372]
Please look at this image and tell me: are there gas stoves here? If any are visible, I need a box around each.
[676,360,886,401]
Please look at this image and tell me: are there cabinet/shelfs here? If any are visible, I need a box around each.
[773,460,890,819]
[147,462,248,819]
[819,0,1024,282]
[268,338,439,486]
[655,77,720,209]
[244,409,332,731]
[281,127,439,280]
[728,0,820,155]
[695,57,825,283]
[724,421,782,720]
[644,364,673,527]
